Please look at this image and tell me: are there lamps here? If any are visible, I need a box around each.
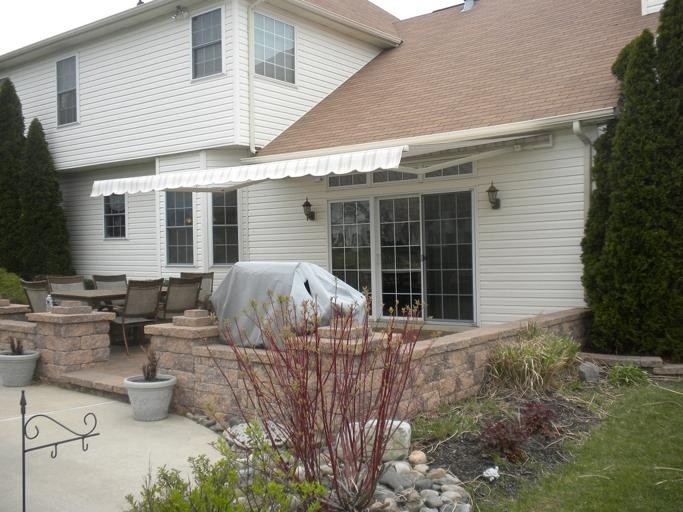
[486,179,501,210]
[301,196,315,221]
[168,6,186,24]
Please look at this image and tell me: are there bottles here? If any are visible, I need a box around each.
[45,295,53,312]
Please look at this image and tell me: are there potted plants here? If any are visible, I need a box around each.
[0,334,40,388]
[125,350,176,420]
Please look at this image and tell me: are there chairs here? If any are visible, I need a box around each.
[19,272,216,357]
